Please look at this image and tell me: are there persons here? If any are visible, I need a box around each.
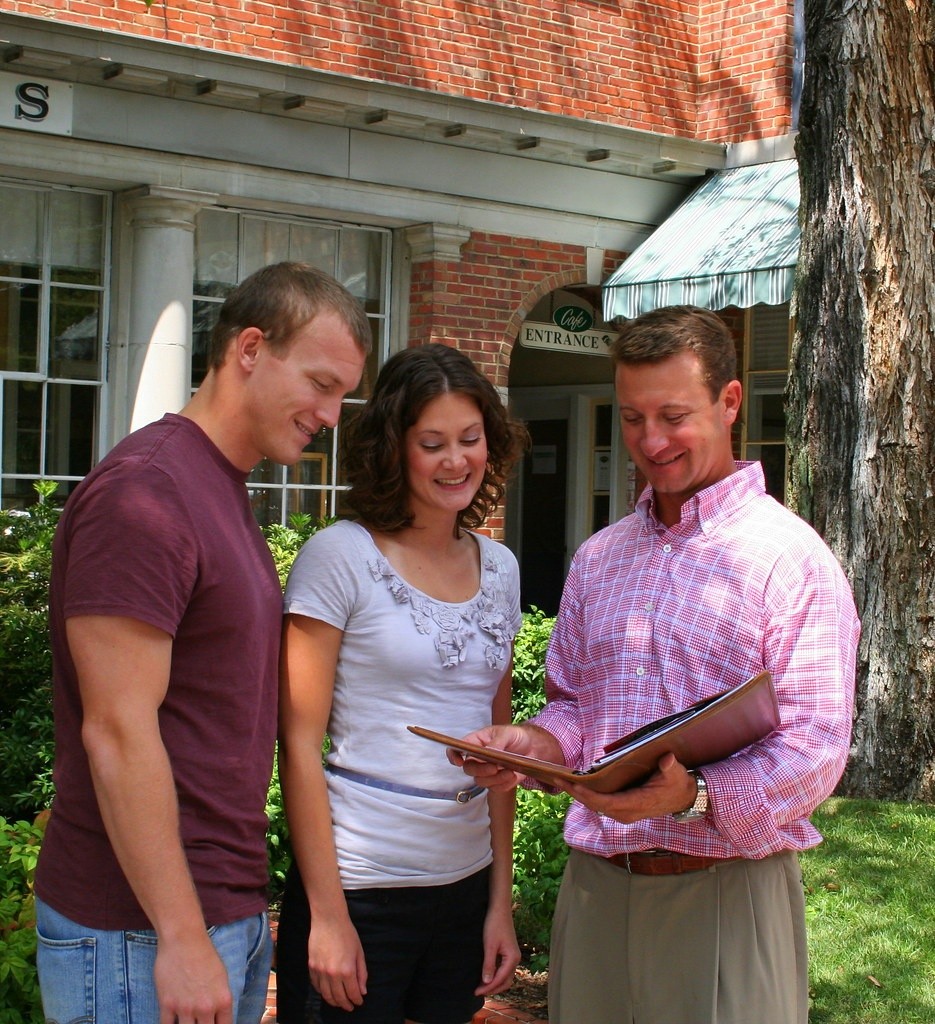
[32,262,371,1024]
[276,340,522,1024]
[445,303,864,1024]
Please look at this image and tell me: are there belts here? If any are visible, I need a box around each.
[325,763,486,804]
[606,847,743,875]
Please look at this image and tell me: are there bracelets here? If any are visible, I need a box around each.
[671,770,709,826]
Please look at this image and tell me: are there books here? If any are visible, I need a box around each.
[408,670,783,791]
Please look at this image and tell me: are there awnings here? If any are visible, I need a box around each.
[598,130,800,321]
